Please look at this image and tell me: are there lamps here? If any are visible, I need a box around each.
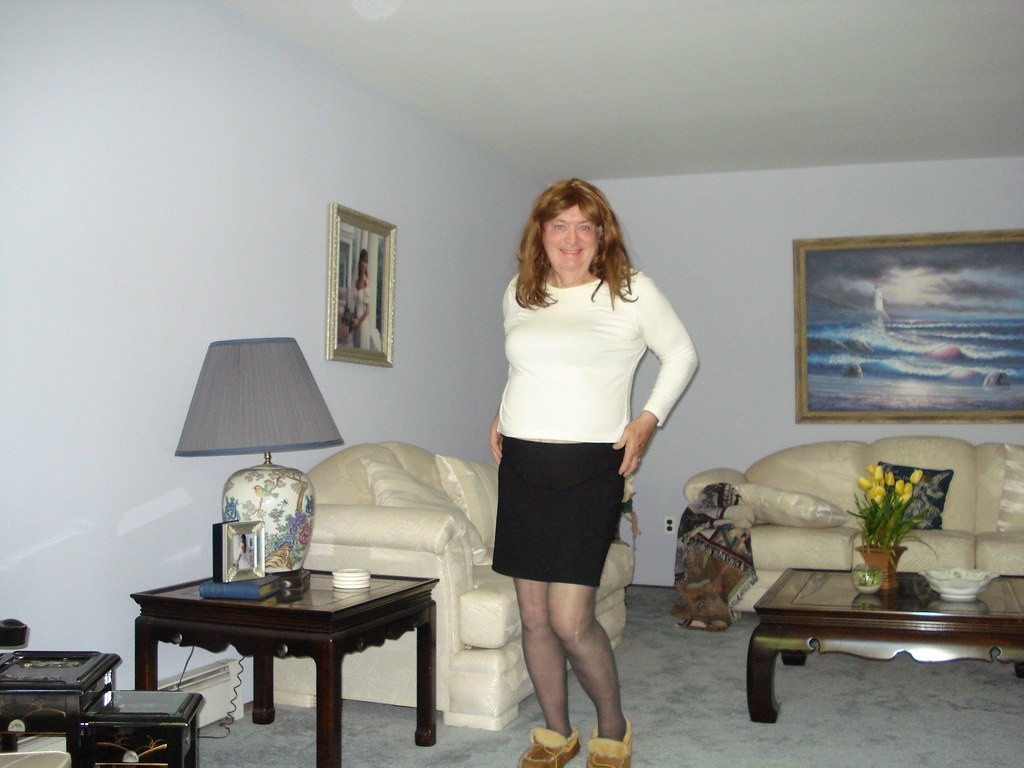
[173,337,344,584]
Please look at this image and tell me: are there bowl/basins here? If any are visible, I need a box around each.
[918,569,1000,598]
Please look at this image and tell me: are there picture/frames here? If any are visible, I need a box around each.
[325,202,397,369]
[792,228,1024,425]
[222,521,265,583]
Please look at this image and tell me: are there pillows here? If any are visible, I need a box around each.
[733,483,849,528]
[360,457,493,566]
[435,453,499,547]
[878,462,954,530]
[997,443,1024,533]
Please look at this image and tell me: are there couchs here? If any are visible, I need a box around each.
[672,434,1024,632]
[272,441,635,731]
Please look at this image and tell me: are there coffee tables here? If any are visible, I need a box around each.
[746,567,1024,724]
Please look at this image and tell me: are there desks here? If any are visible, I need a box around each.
[130,569,440,768]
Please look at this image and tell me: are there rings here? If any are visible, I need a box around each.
[633,457,641,462]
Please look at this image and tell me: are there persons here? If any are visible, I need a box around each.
[234,534,251,570]
[351,249,381,352]
[489,177,700,768]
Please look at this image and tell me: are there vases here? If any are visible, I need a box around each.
[856,545,908,590]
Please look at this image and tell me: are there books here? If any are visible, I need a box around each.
[199,575,280,601]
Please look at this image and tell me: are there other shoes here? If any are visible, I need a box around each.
[519,718,632,768]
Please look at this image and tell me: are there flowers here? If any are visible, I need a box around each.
[846,465,939,570]
